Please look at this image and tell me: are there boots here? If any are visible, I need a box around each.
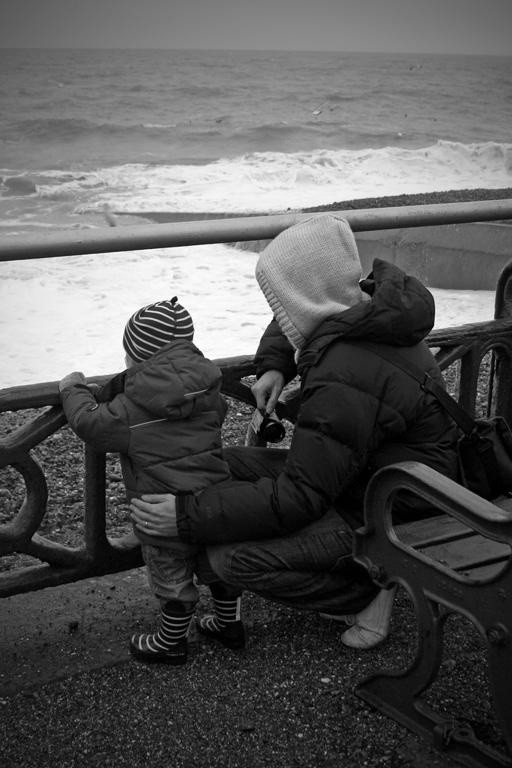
[196,590,246,653]
[128,605,192,666]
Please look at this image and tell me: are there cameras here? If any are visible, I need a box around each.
[251,406,285,442]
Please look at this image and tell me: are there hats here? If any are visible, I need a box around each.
[121,295,197,367]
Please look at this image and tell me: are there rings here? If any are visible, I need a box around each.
[145,521,148,528]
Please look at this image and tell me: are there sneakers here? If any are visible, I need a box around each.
[317,581,400,653]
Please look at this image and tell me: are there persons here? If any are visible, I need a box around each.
[129,212,481,652]
[59,294,248,664]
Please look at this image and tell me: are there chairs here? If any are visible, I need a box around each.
[349,453,512,762]
